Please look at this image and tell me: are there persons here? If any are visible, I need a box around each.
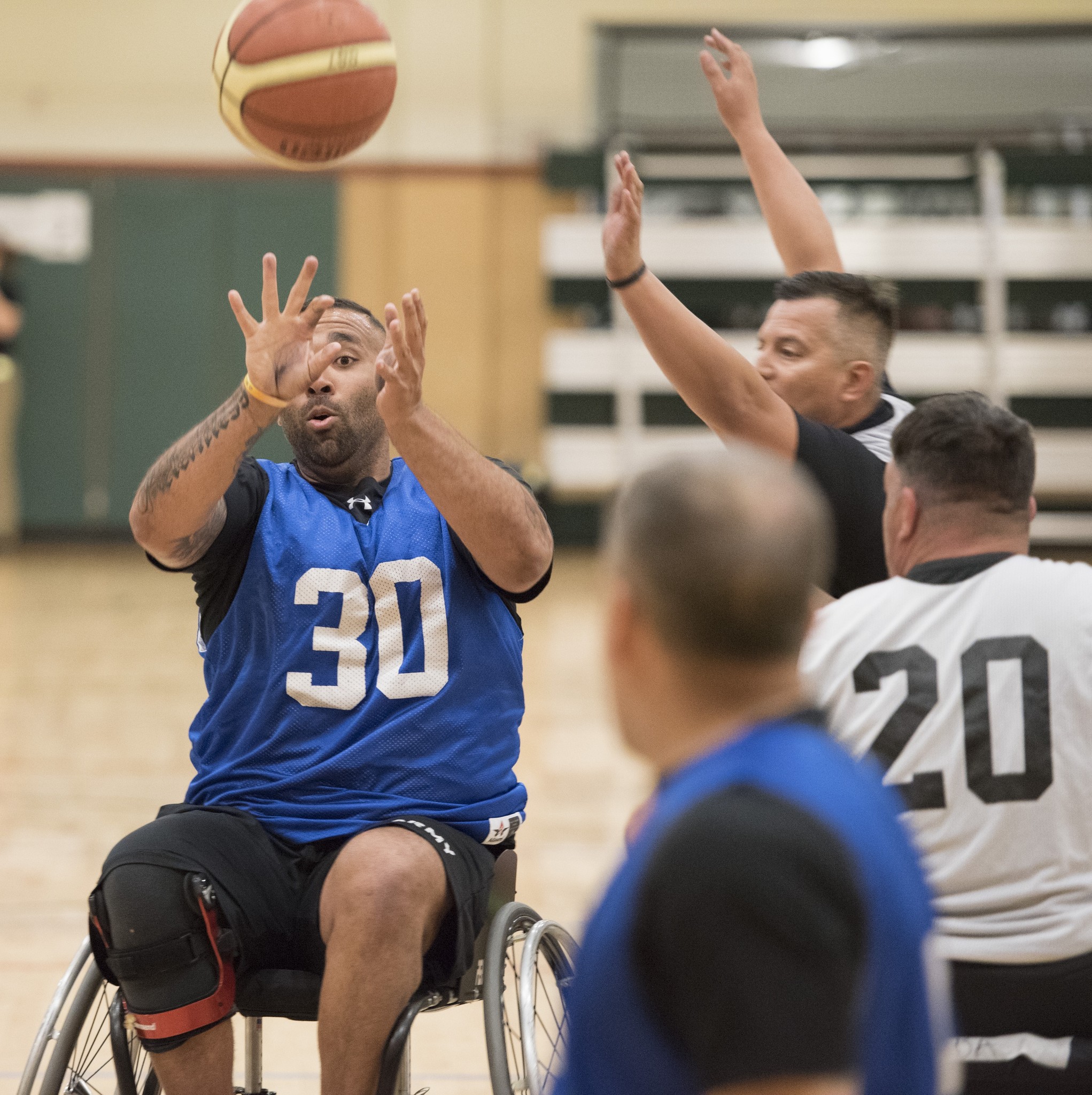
[599,24,919,600]
[91,252,555,1095]
[786,389,1092,1094]
[533,443,967,1094]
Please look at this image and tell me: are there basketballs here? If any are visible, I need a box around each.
[212,1,398,170]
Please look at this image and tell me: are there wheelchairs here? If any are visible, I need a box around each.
[13,798,586,1095]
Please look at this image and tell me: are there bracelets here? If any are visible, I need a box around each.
[605,264,655,290]
[241,372,294,411]
